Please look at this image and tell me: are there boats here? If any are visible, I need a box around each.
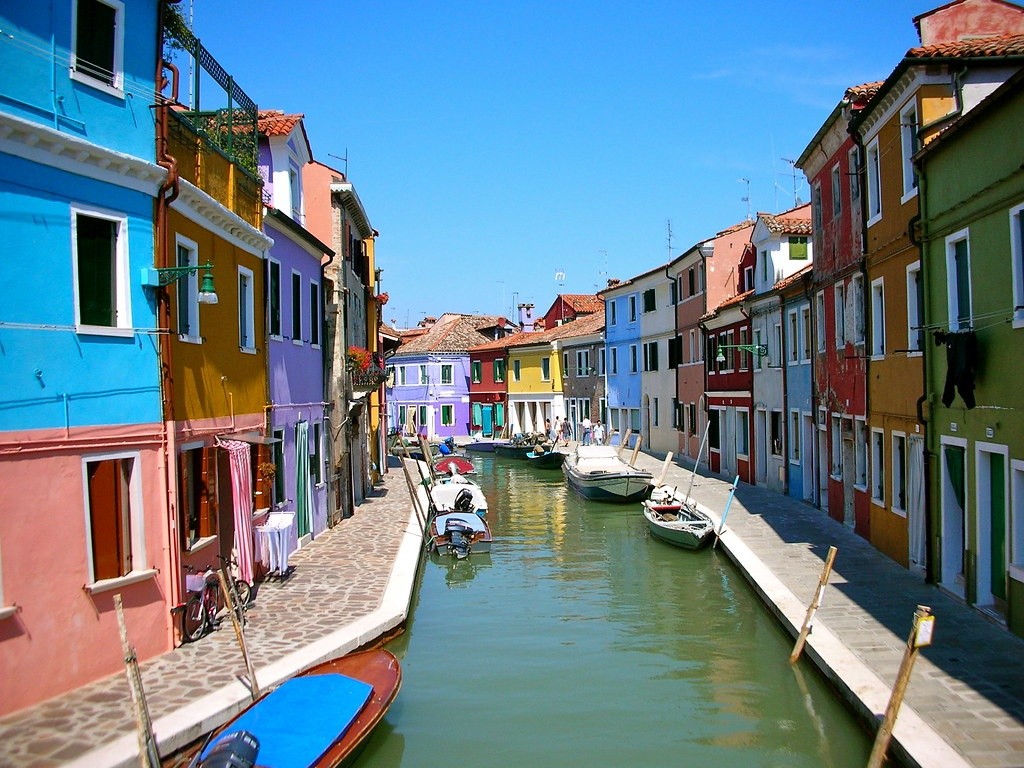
[464,435,555,460]
[392,428,495,561]
[641,487,715,551]
[562,444,655,502]
[174,650,404,768]
[526,451,570,470]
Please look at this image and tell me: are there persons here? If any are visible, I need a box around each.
[545,415,606,446]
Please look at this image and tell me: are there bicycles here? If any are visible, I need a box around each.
[181,554,252,642]
